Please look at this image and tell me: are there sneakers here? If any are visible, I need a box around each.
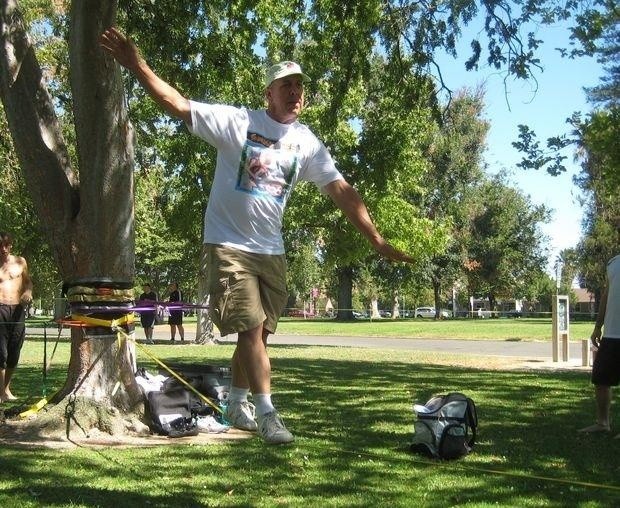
[194,414,230,433]
[142,339,184,344]
[168,417,199,437]
[223,400,258,431]
[257,408,294,445]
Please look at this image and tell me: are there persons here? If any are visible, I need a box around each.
[96,20,418,447]
[168,282,186,345]
[575,252,620,435]
[0,229,34,406]
[139,283,159,345]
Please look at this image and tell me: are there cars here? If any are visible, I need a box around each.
[288,305,524,320]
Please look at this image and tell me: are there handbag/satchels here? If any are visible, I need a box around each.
[409,393,478,463]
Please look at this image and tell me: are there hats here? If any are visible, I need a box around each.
[142,283,150,286]
[265,60,312,90]
[170,282,178,286]
